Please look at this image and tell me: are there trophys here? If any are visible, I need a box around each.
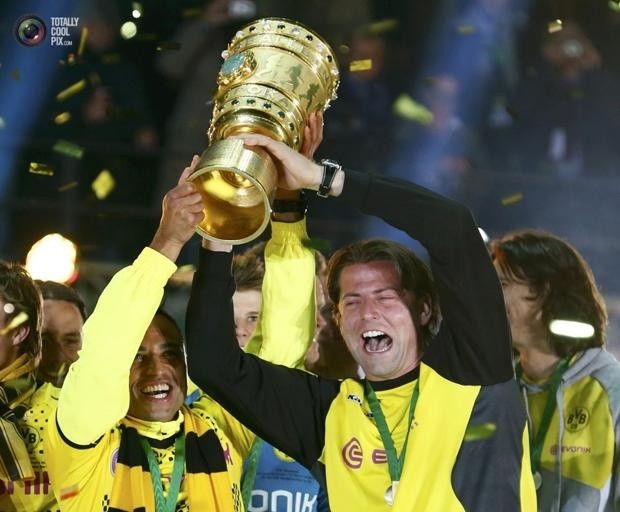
[185,17,340,246]
